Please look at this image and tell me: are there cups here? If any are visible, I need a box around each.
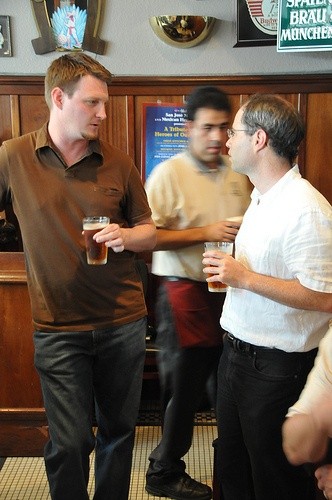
[203,241,235,292]
[83,217,109,264]
[227,217,243,230]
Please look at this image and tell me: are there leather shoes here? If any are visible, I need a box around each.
[145,474,212,500]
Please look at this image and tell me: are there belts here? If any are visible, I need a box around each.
[226,333,272,355]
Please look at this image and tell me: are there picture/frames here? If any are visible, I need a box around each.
[0,15,12,57]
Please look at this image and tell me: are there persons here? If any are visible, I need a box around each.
[142,86,252,500]
[281,325,332,500]
[201,95,332,500]
[0,24,6,55]
[0,52,154,500]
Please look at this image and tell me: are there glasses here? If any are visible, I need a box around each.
[227,128,267,146]
